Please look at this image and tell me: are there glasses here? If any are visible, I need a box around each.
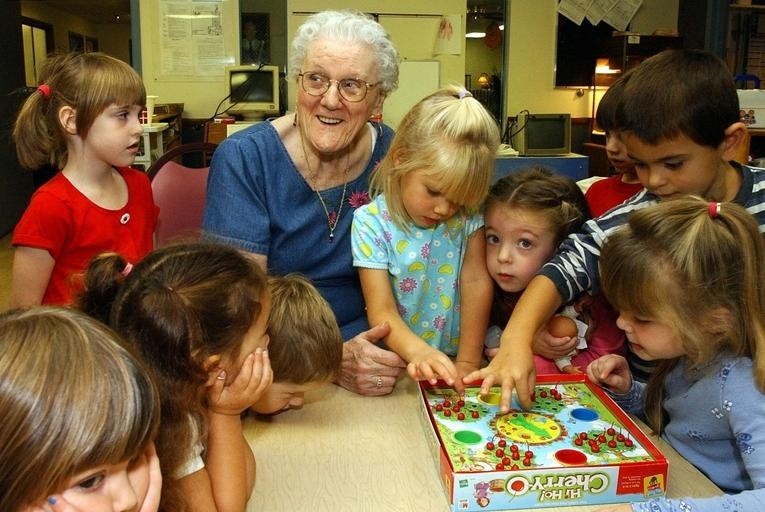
[298,70,383,103]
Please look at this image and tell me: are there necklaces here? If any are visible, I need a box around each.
[293,113,357,243]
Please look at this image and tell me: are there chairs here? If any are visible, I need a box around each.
[142,141,219,247]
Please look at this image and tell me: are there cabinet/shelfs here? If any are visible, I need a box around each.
[139,112,182,166]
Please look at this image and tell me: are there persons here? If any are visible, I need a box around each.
[587,194,765,511]
[462,48,764,415]
[585,64,642,218]
[248,272,343,415]
[77,240,274,512]
[547,296,590,376]
[0,306,162,512]
[485,167,627,374]
[351,86,501,396]
[8,51,162,309]
[200,9,406,396]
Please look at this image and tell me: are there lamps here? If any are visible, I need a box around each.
[478,72,489,84]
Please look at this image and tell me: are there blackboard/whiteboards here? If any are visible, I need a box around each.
[380,58,441,133]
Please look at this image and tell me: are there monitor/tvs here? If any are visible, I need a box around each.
[511,113,571,155]
[225,66,279,123]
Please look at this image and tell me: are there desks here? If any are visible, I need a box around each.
[243,369,731,512]
